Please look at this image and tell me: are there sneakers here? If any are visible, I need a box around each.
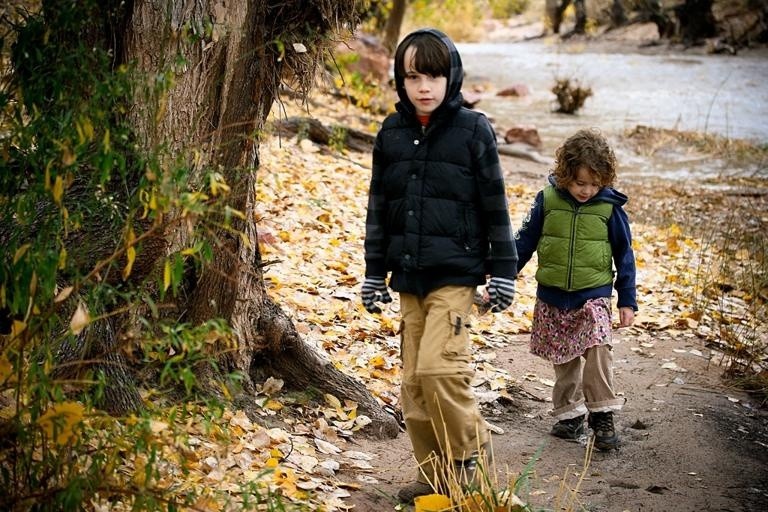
[399,459,482,506]
[552,414,585,438]
[588,412,617,450]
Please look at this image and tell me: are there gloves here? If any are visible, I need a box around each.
[361,276,392,314]
[484,277,514,312]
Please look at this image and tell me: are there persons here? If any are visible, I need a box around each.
[358,27,520,505]
[514,126,640,454]
